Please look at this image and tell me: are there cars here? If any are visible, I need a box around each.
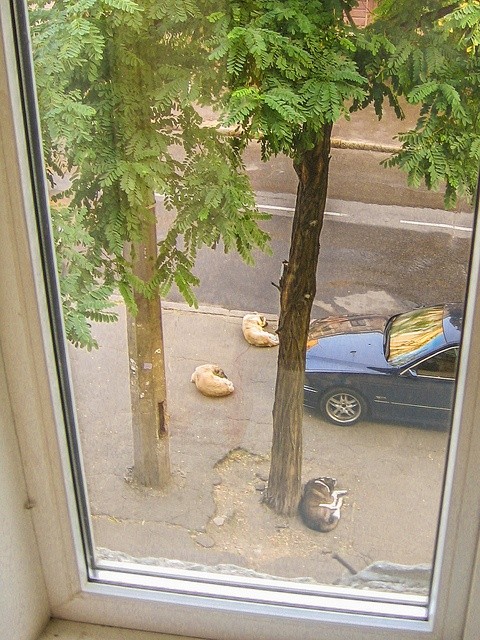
[303,302,464,433]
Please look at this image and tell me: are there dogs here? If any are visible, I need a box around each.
[191,364,234,397]
[298,476,348,533]
[242,312,279,348]
